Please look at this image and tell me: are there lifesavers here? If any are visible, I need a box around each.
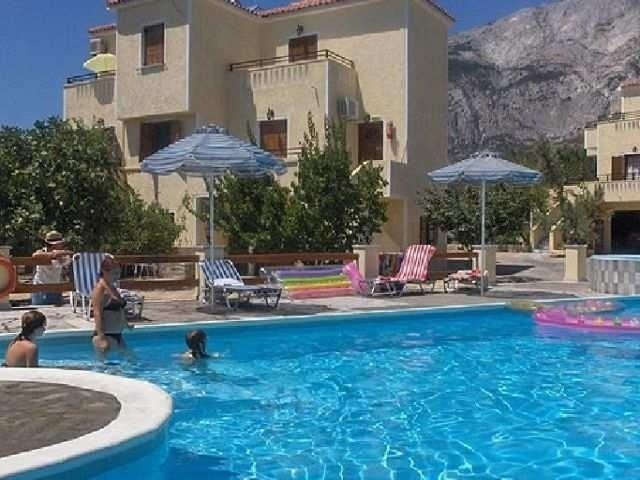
[0,256,16,296]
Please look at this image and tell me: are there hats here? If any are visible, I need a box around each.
[44,231,65,245]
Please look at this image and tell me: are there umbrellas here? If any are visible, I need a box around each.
[140,125,287,306]
[430,152,542,292]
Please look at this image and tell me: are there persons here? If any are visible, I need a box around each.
[180,314,217,370]
[91,256,137,371]
[31,231,73,304]
[5,309,45,367]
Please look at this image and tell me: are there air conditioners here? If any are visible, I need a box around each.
[339,96,361,121]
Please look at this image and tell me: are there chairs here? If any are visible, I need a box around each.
[444,268,489,295]
[70,251,144,323]
[368,243,438,297]
[199,259,280,310]
